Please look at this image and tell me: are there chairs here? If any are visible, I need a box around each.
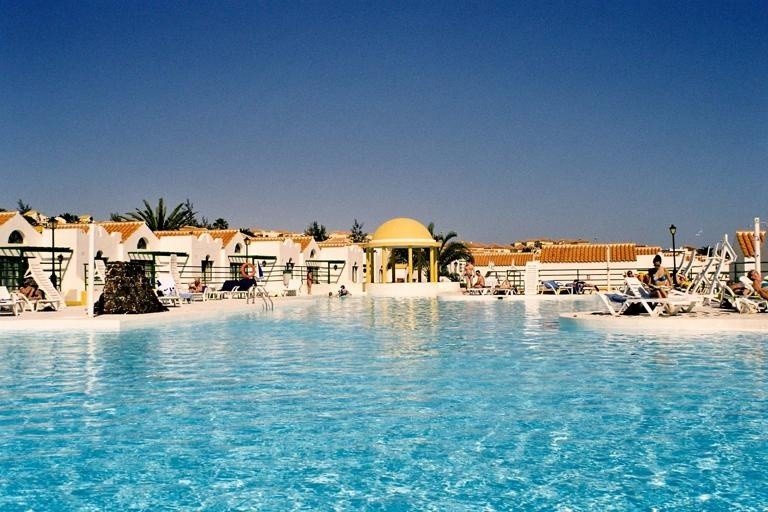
[466,274,596,296]
[595,276,768,318]
[282,279,301,296]
[0,286,60,316]
[157,284,255,307]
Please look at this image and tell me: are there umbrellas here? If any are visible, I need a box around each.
[255,260,264,283]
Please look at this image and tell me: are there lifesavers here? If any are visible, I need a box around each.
[241,263,255,279]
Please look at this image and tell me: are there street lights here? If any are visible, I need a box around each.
[47,216,65,295]
[669,224,677,287]
[243,236,250,279]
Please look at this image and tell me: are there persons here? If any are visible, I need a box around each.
[328,292,333,298]
[463,260,475,294]
[623,254,768,316]
[18,278,46,302]
[499,279,516,290]
[470,269,486,288]
[572,279,600,293]
[338,284,351,297]
[306,270,314,296]
[188,278,202,293]
